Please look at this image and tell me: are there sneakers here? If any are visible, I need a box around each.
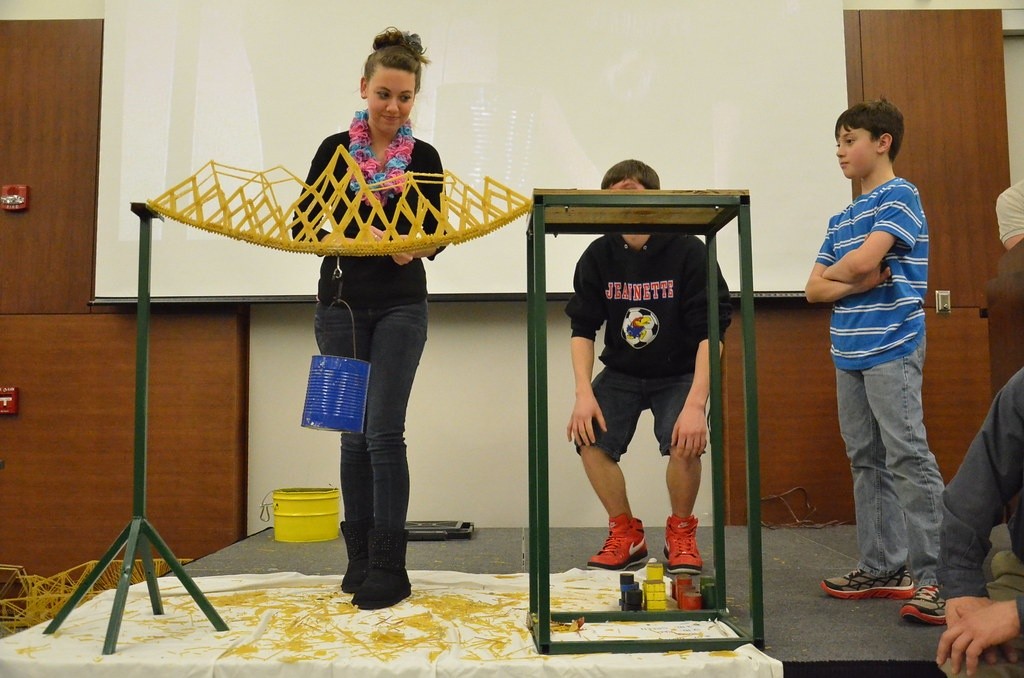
[900,584,946,625]
[820,568,916,600]
[663,515,703,574]
[586,514,649,571]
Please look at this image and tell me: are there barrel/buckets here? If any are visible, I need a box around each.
[301,299,370,433]
[259,487,340,543]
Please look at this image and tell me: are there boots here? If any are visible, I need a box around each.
[351,528,412,610]
[340,518,375,593]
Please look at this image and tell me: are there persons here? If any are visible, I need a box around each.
[291,25,443,608]
[936,366,1023,678]
[995,178,1024,249]
[565,159,733,576]
[805,97,946,627]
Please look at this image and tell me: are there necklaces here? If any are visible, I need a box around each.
[347,108,414,207]
